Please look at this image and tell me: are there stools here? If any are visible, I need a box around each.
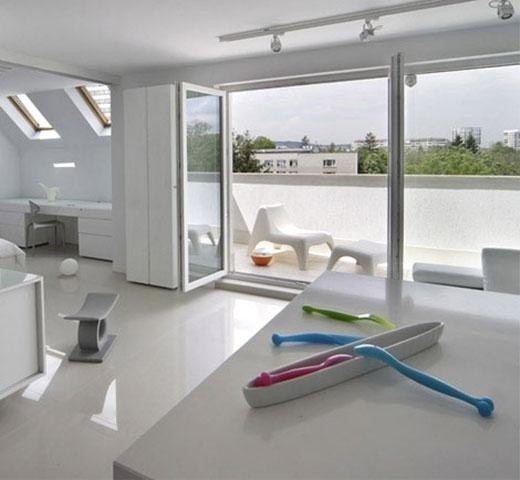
[63,292,120,362]
[328,241,388,277]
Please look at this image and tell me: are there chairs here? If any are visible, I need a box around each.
[481,247,519,292]
[248,203,335,270]
[25,201,66,255]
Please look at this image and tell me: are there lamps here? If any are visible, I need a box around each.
[218,1,514,53]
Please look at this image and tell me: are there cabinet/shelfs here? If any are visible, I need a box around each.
[1,269,47,400]
[0,198,112,261]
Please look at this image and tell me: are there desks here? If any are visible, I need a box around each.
[113,269,520,479]
